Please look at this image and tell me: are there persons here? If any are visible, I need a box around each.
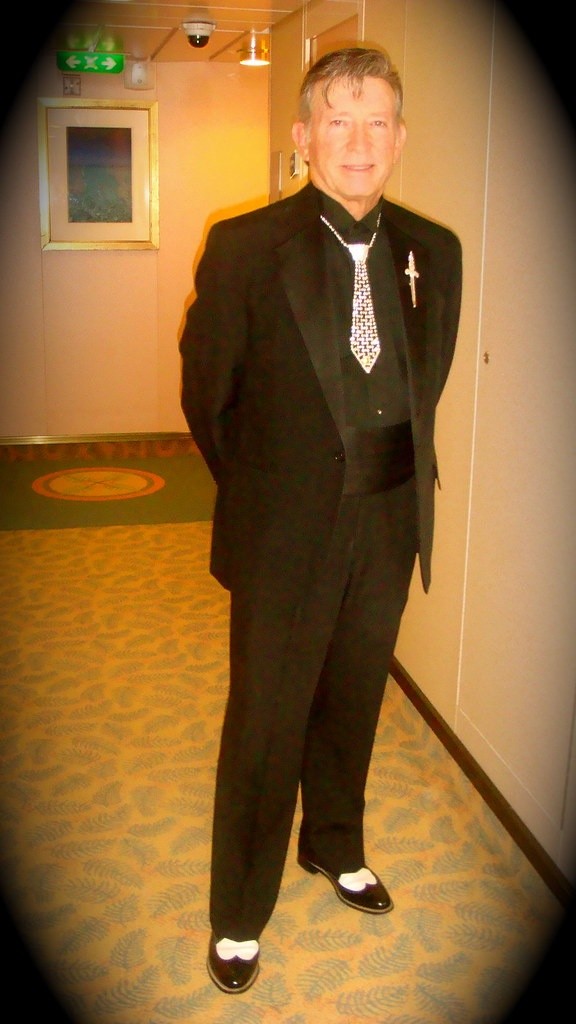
[177,48,463,993]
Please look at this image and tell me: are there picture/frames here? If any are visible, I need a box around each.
[36,97,160,251]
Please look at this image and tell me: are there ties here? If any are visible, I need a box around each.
[319,212,384,376]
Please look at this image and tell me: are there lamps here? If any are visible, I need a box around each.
[236,31,272,66]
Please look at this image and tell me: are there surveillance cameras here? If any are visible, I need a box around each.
[183,22,215,47]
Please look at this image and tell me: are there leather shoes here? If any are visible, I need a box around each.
[206,928,261,994]
[297,853,394,916]
